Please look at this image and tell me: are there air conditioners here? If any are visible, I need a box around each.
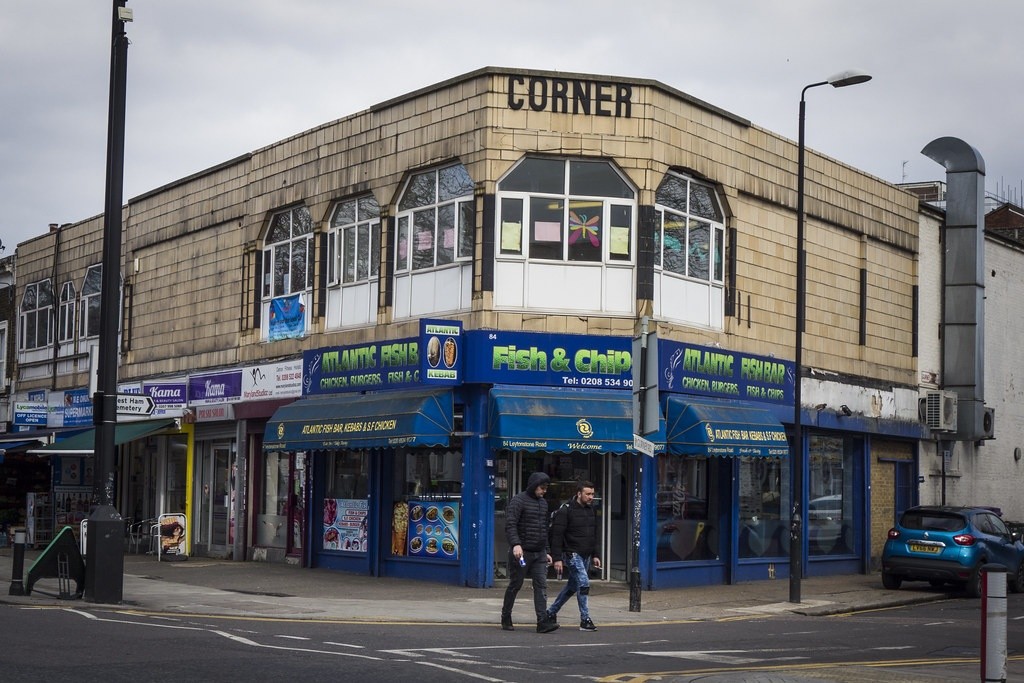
[926,390,958,433]
[983,407,995,439]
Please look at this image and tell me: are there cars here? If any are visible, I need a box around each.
[880,505,1023,596]
[802,494,846,549]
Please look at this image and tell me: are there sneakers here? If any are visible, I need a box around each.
[579,617,596,632]
[501,614,515,631]
[536,620,560,633]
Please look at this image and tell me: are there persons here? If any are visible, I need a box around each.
[500,472,561,634]
[545,478,602,631]
[160,522,186,553]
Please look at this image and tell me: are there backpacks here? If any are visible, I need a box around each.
[549,502,572,551]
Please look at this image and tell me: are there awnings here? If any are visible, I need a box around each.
[26,417,175,454]
[659,393,790,463]
[262,386,456,454]
[0,441,37,465]
[486,386,668,459]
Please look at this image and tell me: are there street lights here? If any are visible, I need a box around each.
[790,71,870,604]
